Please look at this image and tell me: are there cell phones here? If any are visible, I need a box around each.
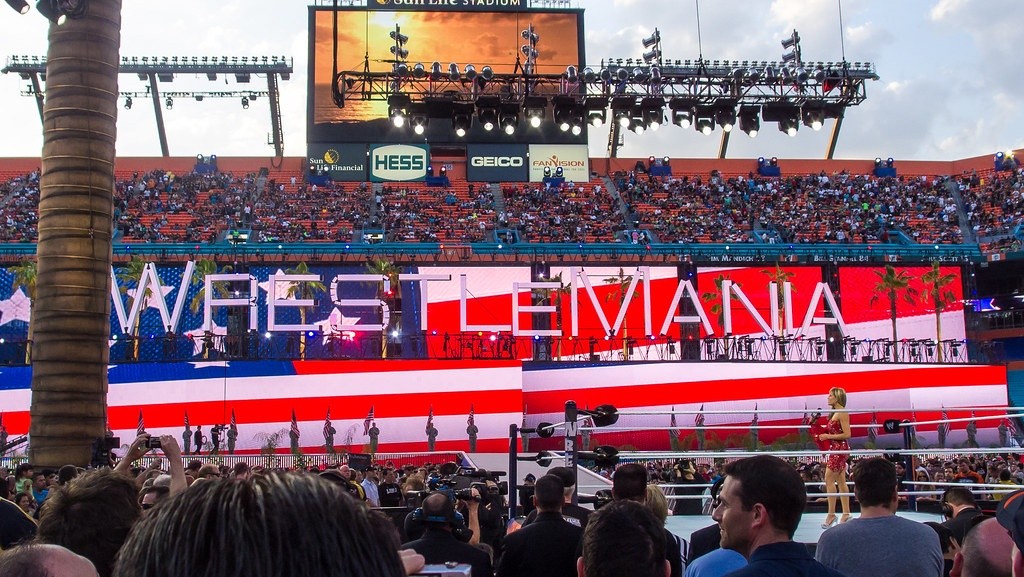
[145,436,161,448]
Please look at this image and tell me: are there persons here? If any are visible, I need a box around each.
[1,168,1024,577]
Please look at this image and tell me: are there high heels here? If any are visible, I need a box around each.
[821,514,838,529]
[839,513,852,523]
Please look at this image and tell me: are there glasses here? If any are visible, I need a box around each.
[208,473,220,477]
[142,504,154,510]
[919,476,926,478]
[1007,530,1024,555]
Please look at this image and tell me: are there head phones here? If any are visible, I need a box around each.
[412,508,464,529]
[941,486,981,518]
[320,470,360,500]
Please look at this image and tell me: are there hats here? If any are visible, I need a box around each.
[996,490,1024,555]
[365,466,377,472]
[547,467,575,487]
[251,466,262,473]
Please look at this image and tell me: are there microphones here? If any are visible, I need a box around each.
[809,408,821,425]
[490,471,506,476]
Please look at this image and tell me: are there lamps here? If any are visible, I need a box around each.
[165,97,173,109]
[124,97,132,109]
[240,98,249,109]
[389,30,824,138]
